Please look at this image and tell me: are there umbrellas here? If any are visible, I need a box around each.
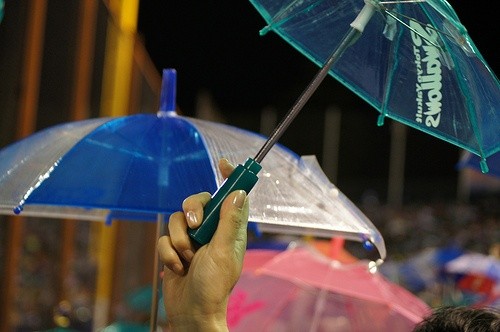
[248,226,500,300]
[183,0,500,250]
[226,235,435,332]
[1,70,380,332]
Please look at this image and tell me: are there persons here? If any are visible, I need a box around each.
[157,156,500,332]
